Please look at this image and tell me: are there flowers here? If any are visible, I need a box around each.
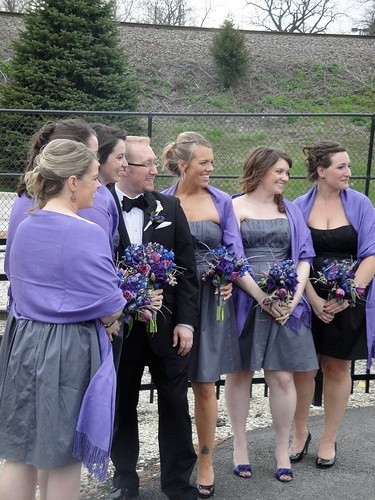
[253,243,301,324]
[113,242,187,334]
[195,240,265,323]
[308,256,371,308]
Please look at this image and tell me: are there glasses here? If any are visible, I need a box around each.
[127,160,160,168]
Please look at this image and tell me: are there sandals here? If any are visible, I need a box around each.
[234,463,252,478]
[197,482,215,498]
[275,467,293,482]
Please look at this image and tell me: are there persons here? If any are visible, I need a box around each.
[0,119,127,500]
[289,142,375,466]
[90,121,129,189]
[227,145,317,482]
[160,131,244,498]
[107,134,197,500]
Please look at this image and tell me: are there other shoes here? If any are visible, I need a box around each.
[168,484,199,500]
[107,486,139,500]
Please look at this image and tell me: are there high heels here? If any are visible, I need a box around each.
[290,429,311,462]
[315,441,337,468]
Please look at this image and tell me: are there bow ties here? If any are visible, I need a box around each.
[121,194,149,213]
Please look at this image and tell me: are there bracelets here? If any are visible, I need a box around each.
[102,321,115,329]
